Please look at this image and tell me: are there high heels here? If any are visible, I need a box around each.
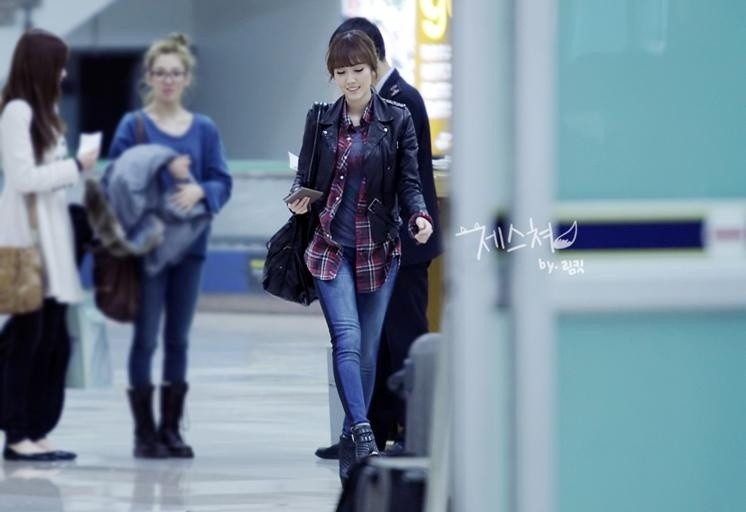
[339,427,380,485]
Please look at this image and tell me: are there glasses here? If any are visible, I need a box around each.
[151,69,184,81]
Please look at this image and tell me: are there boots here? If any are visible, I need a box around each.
[127,382,195,459]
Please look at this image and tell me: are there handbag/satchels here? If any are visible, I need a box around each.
[0,246,44,314]
[92,250,140,321]
[262,213,319,305]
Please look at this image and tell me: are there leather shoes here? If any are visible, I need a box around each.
[315,443,339,460]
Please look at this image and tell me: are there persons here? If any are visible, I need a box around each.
[288,18,443,490]
[0,29,101,461]
[101,33,233,458]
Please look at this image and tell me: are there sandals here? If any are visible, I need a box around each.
[4,446,76,463]
